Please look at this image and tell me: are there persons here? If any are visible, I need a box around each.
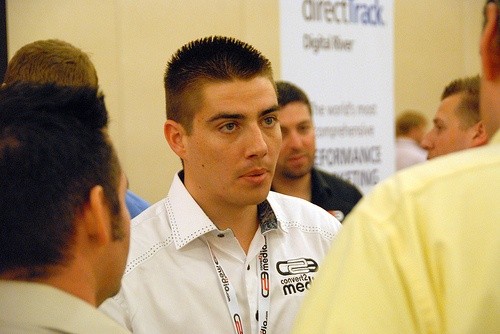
[267,80,363,224]
[99,35,344,334]
[420,74,485,160]
[286,0,500,334]
[2,39,151,219]
[393,109,429,172]
[0,85,131,334]
